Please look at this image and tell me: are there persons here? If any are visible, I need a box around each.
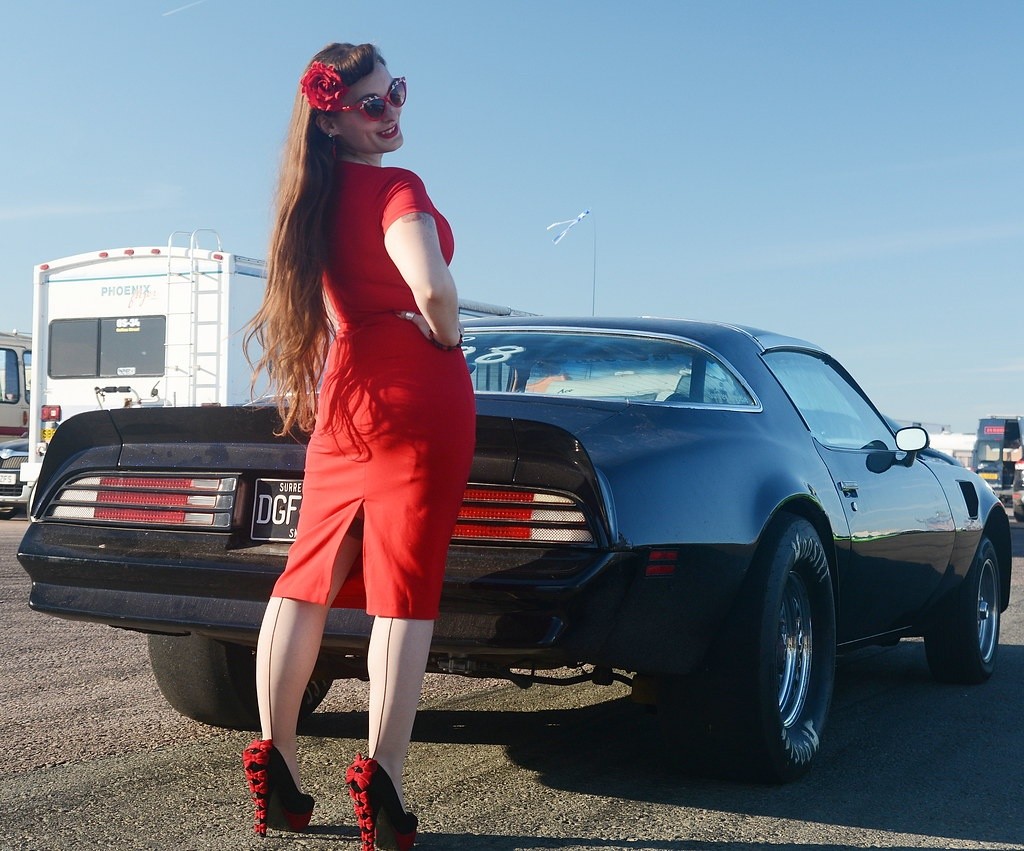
[230,40,476,851]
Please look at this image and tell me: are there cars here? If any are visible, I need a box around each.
[1011,457,1024,522]
[0,431,32,519]
[14,316,1011,786]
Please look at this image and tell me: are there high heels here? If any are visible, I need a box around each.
[242,739,315,837]
[345,753,418,851]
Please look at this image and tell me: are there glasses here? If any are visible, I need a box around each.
[337,76,407,122]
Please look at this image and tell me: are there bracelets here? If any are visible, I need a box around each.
[428,327,462,352]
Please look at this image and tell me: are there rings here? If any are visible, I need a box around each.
[405,311,417,321]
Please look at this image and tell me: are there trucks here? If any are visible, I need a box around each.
[19,227,555,489]
[970,415,1024,505]
[0,330,33,451]
